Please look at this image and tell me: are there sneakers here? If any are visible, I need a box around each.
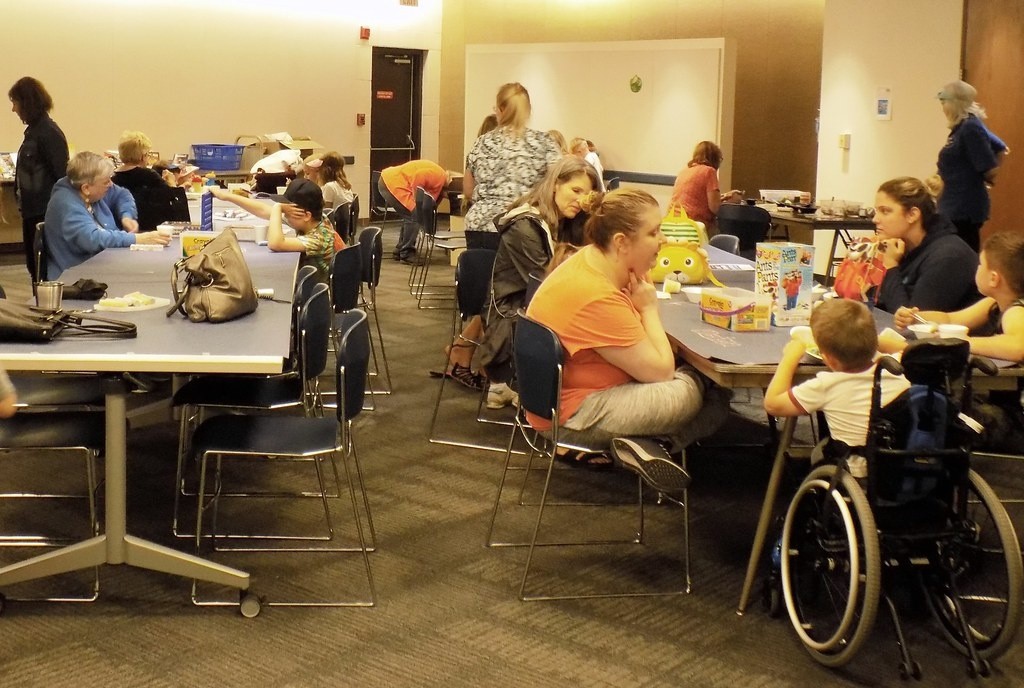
[487,382,523,409]
[393,255,425,266]
[610,438,691,493]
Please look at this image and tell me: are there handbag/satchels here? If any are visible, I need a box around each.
[167,226,258,323]
[0,298,137,343]
[833,238,887,303]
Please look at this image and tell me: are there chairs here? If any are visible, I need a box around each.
[171,170,404,608]
[709,235,741,254]
[718,203,772,262]
[0,284,132,518]
[407,186,700,600]
[0,409,130,604]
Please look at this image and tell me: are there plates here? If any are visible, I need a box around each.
[94,297,171,311]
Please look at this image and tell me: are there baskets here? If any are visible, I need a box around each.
[191,142,246,171]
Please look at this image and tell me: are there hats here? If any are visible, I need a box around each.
[269,179,322,203]
[306,159,323,168]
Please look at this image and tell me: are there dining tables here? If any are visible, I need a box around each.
[653,243,1024,619]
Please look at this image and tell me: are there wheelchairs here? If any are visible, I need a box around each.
[763,338,1024,681]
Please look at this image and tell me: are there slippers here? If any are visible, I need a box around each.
[430,364,488,390]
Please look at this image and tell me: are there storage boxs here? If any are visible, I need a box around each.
[192,144,245,170]
[754,243,815,327]
[760,189,812,205]
[179,230,221,258]
[698,288,772,332]
[248,137,324,160]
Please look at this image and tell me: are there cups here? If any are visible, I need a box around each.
[277,186,286,195]
[157,225,173,247]
[192,176,203,193]
[253,225,268,244]
[206,174,215,186]
[747,199,756,206]
[662,279,681,293]
[35,281,65,308]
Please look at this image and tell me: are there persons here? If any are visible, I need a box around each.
[378,159,450,266]
[871,177,999,337]
[935,80,1011,255]
[286,151,355,236]
[429,82,612,470]
[111,131,190,234]
[762,298,911,552]
[7,76,70,296]
[45,151,172,281]
[208,178,347,288]
[894,230,1024,365]
[524,187,729,493]
[667,140,741,241]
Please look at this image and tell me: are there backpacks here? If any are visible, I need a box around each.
[647,202,727,288]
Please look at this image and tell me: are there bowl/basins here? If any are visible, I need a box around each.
[907,324,969,340]
[821,199,864,217]
[813,288,827,301]
[680,287,701,303]
[793,206,818,213]
[790,326,823,361]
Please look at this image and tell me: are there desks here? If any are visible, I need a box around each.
[741,199,876,286]
[0,191,301,619]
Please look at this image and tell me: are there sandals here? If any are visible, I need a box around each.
[556,450,614,470]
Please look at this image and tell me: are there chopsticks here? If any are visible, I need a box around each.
[901,305,930,326]
